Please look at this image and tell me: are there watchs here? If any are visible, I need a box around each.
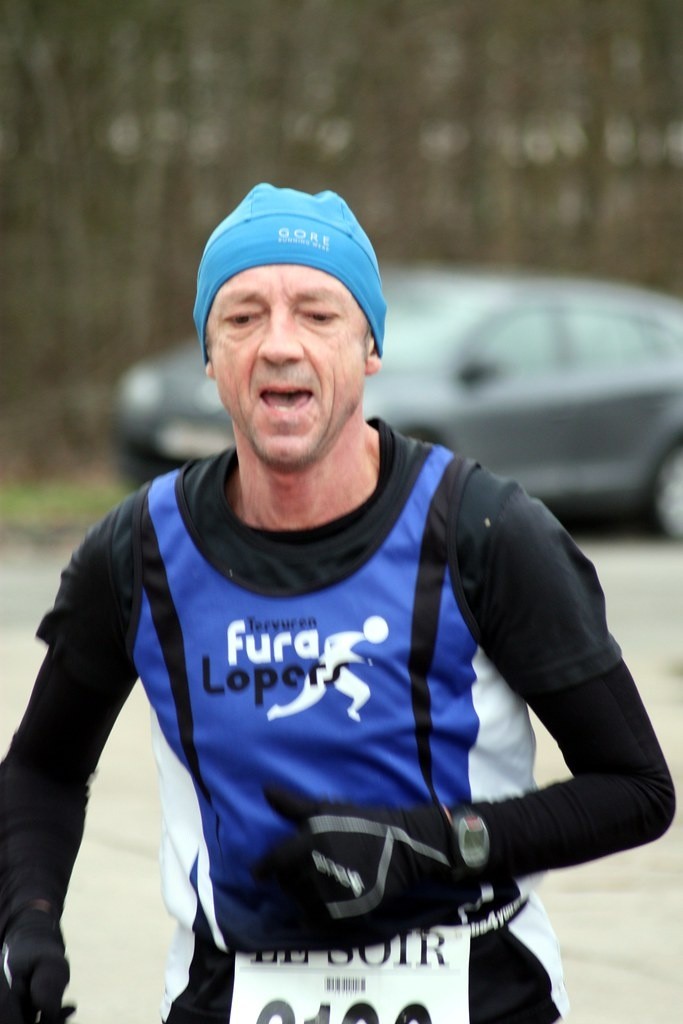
[449,800,490,881]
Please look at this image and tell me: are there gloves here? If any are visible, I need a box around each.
[261,779,464,934]
[0,908,75,1024]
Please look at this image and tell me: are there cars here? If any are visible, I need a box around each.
[107,254,682,554]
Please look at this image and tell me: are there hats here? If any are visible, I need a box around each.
[192,180,388,365]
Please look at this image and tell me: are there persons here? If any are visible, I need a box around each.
[1,183,677,1024]
